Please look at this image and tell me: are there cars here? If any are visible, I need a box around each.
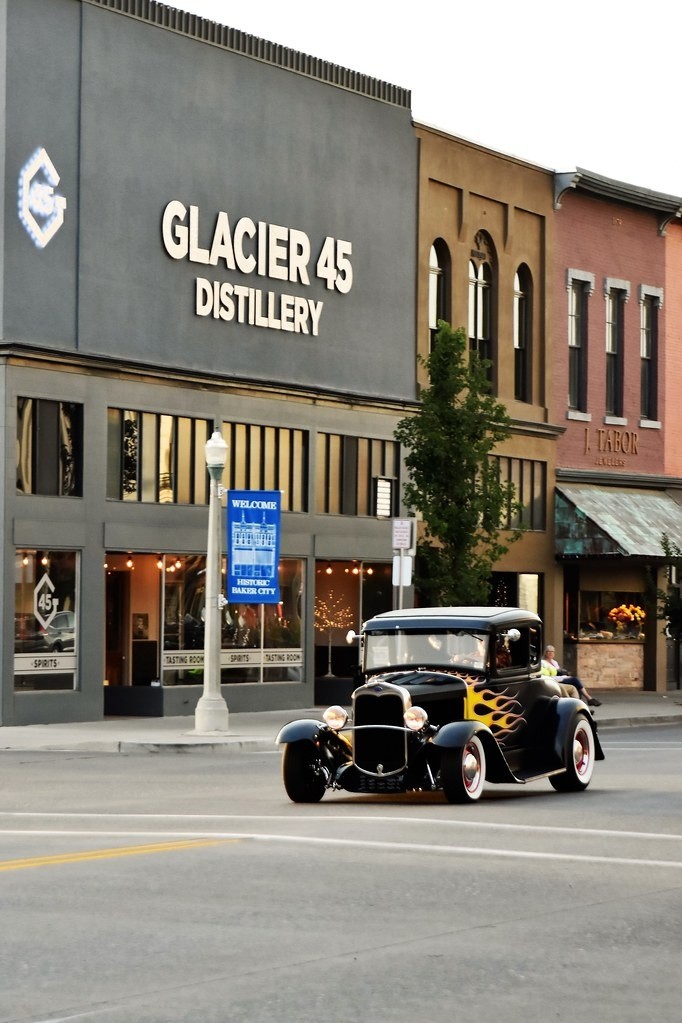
[274,606,606,804]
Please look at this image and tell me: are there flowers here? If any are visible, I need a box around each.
[608,603,647,625]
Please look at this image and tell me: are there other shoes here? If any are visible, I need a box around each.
[588,698,601,706]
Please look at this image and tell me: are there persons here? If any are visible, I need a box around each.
[540,646,602,715]
[450,633,511,672]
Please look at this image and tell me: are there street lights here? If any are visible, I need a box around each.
[192,428,233,736]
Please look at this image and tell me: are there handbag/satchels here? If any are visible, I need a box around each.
[557,668,568,675]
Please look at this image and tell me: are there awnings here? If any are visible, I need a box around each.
[553,485,682,560]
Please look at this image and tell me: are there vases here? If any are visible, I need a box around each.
[614,625,642,638]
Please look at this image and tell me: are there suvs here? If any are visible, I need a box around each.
[14,611,75,655]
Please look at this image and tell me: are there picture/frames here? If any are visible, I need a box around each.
[132,613,149,639]
